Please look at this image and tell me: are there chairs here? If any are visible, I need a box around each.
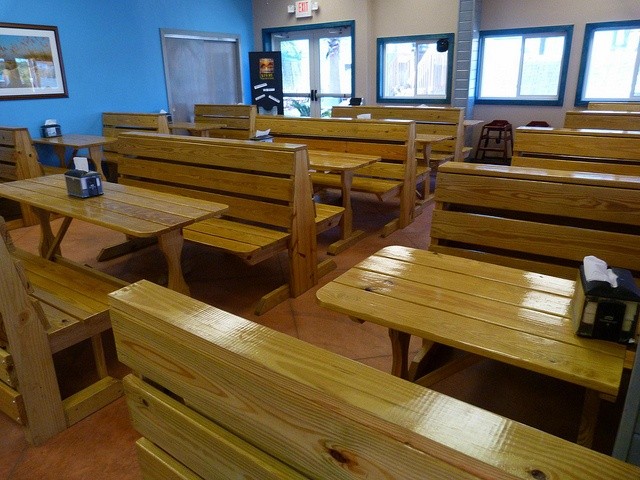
[316,242,635,451]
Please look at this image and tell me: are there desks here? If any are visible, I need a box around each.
[304,143,380,255]
[475,116,513,166]
[1,170,229,278]
[415,129,456,206]
[167,116,228,136]
[32,127,119,173]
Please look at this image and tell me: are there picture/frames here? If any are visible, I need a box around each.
[0,21,69,100]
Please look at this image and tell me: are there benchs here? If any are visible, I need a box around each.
[0,125,76,227]
[563,111,640,135]
[257,115,413,237]
[190,97,256,140]
[101,276,639,476]
[99,109,169,164]
[0,217,143,447]
[510,124,637,176]
[115,133,343,314]
[409,160,638,383]
[331,102,461,164]
